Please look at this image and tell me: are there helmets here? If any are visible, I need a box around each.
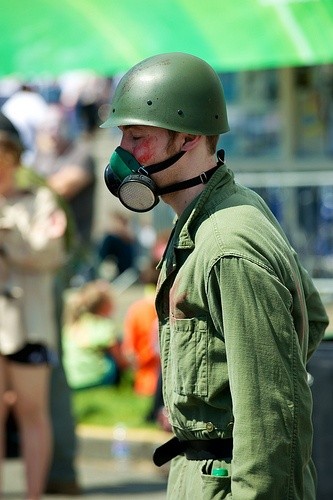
[99,53,229,136]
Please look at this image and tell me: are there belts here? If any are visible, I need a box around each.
[151,434,235,466]
